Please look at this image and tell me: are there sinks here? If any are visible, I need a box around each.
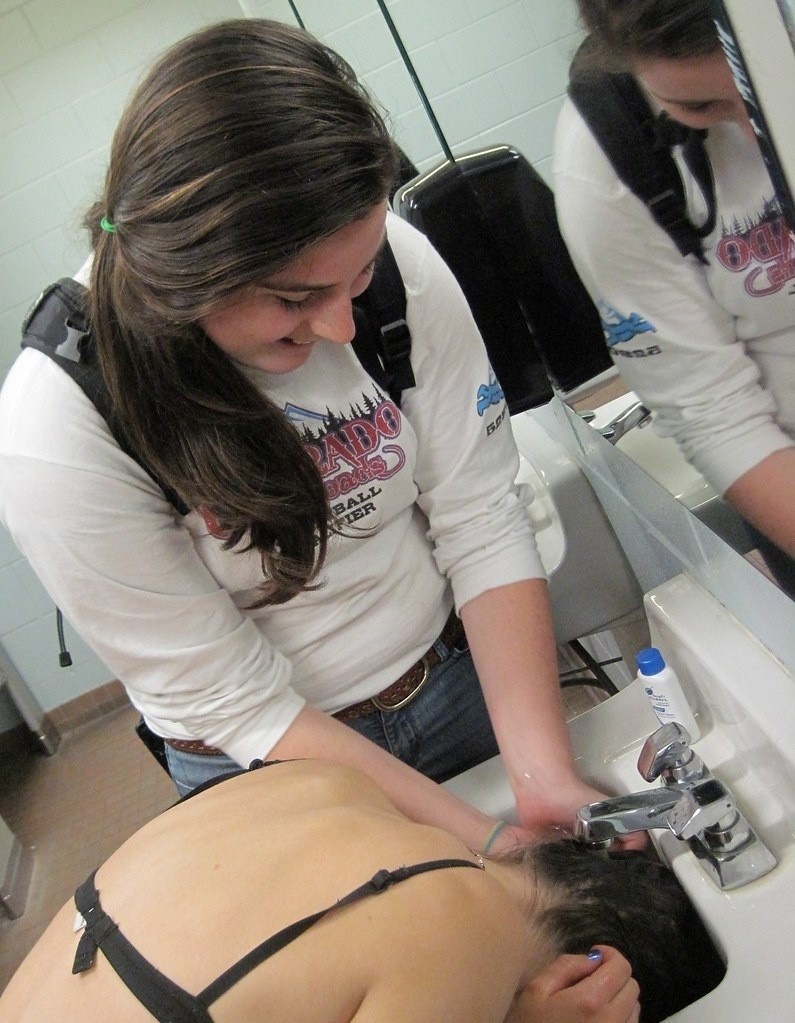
[586,389,717,510]
[434,664,794,1023]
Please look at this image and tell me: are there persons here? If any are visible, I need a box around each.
[1,22,655,855]
[550,0,795,599]
[1,759,726,1023]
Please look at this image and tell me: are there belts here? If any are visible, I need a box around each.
[162,606,466,756]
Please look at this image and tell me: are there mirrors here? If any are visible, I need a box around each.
[284,0,795,679]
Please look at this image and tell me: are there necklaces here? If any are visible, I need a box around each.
[467,847,486,871]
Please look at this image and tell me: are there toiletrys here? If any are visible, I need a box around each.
[635,647,701,746]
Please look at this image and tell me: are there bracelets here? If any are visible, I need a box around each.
[481,819,506,856]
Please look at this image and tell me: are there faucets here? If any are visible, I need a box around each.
[594,398,652,447]
[573,721,778,892]
